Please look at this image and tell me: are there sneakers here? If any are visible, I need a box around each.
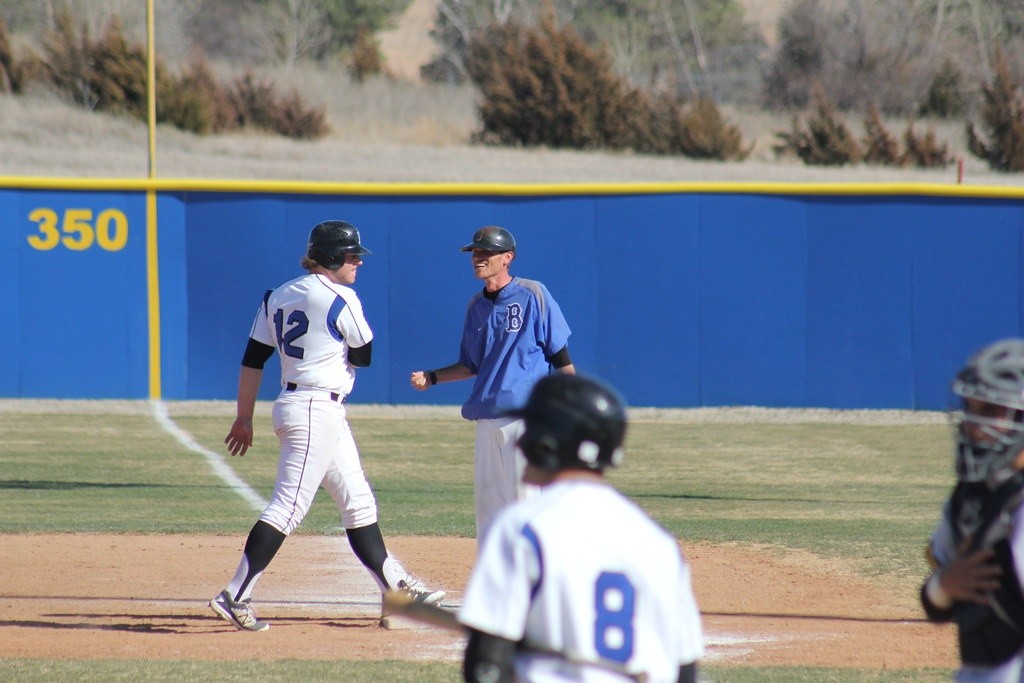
[380,571,448,618]
[207,588,270,632]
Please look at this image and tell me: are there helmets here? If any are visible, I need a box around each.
[515,372,629,473]
[308,220,361,270]
[947,336,1024,483]
[459,226,517,260]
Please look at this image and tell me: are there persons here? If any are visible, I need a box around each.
[208,221,444,632]
[456,374,702,683]
[920,337,1024,682]
[410,225,576,556]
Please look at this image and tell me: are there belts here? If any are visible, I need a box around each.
[286,382,348,404]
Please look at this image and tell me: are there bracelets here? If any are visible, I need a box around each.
[428,370,437,386]
[926,572,950,610]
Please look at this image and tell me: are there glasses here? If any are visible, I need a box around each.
[344,254,360,264]
